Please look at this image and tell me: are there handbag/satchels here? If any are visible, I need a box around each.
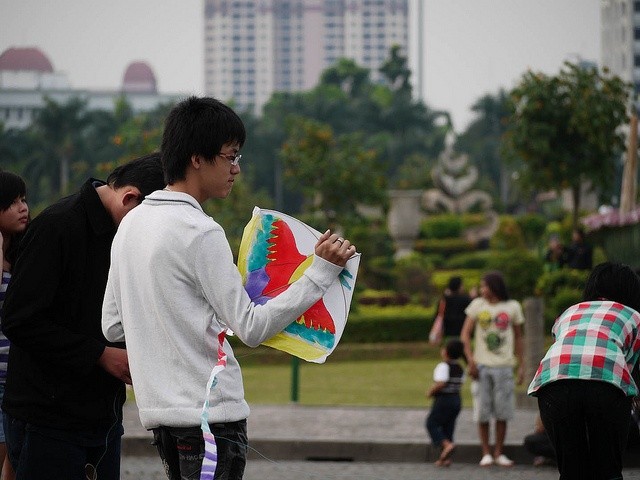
[429,301,446,346]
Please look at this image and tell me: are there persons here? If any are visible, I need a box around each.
[0,171,33,480]
[100,98,357,480]
[526,262,639,479]
[0,152,166,480]
[524,415,560,468]
[428,276,477,346]
[460,271,525,467]
[425,340,474,466]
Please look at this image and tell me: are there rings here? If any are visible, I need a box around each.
[337,238,343,244]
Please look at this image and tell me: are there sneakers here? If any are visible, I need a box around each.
[477,454,495,467]
[496,455,513,466]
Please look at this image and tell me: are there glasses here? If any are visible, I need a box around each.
[214,151,242,166]
